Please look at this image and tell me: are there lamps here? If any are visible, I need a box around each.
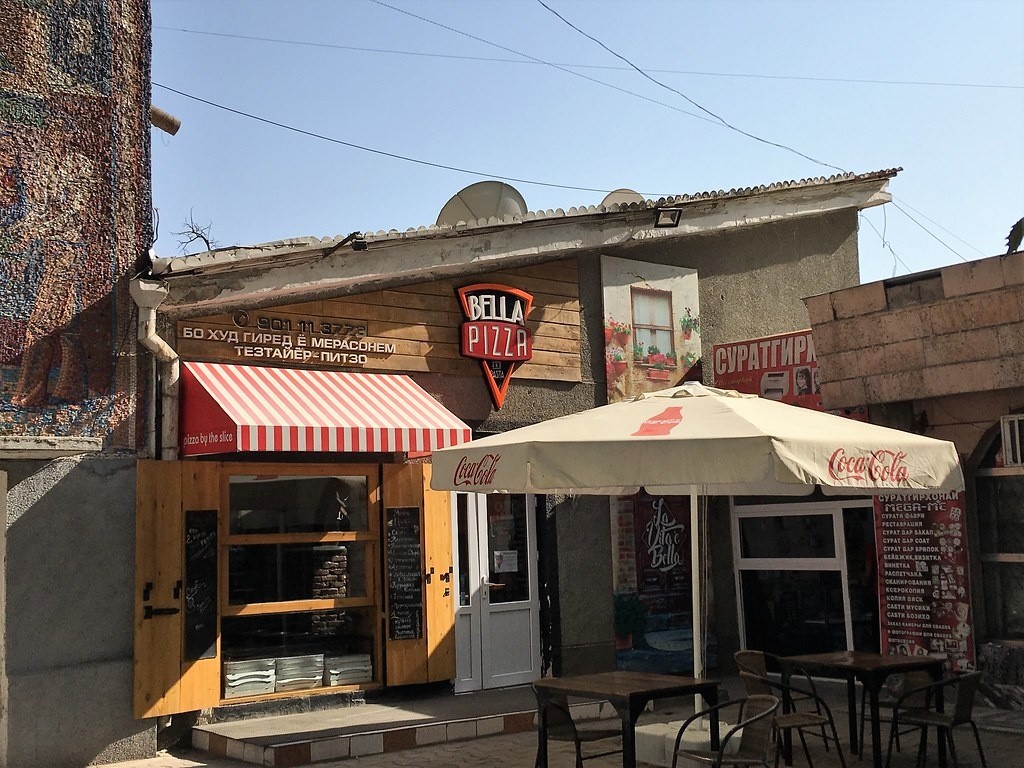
[322,231,368,258]
[654,208,682,227]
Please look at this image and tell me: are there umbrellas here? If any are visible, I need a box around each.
[430,380,964,768]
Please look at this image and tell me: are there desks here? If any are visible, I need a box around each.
[533,671,718,768]
[777,656,948,768]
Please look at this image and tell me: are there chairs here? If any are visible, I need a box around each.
[532,650,988,768]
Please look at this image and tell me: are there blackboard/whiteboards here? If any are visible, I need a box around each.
[184,510,218,660]
[386,507,424,641]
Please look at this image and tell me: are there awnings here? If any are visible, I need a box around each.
[178,361,473,462]
